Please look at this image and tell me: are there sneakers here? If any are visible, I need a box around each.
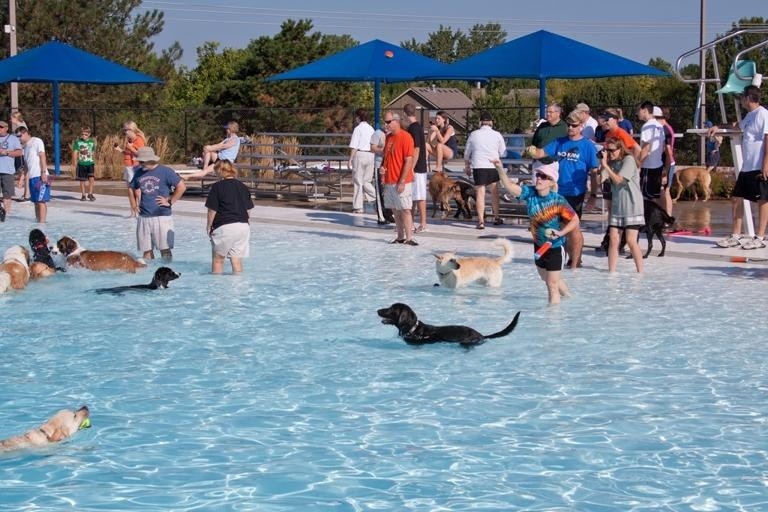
[386,215,396,223]
[81,194,87,200]
[352,209,364,213]
[715,233,741,247]
[377,219,390,224]
[414,226,429,233]
[391,239,406,244]
[15,198,30,202]
[741,235,766,249]
[404,238,418,246]
[88,194,95,200]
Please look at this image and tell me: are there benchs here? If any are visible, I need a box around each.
[427,133,605,226]
[192,131,355,206]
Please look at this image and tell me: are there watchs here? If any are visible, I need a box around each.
[7,151,9,156]
[167,199,173,206]
[41,170,46,173]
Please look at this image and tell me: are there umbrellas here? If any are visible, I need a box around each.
[263,38,490,130]
[450,27,670,121]
[0,37,165,177]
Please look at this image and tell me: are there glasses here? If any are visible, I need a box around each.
[605,118,609,121]
[0,125,4,128]
[17,135,22,137]
[535,172,553,181]
[607,149,618,152]
[567,123,580,128]
[82,134,88,136]
[385,120,391,124]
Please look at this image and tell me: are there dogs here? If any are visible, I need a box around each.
[28,227,64,272]
[429,171,469,220]
[453,180,487,219]
[602,192,675,259]
[431,238,514,290]
[1,404,91,452]
[671,164,714,203]
[376,302,522,350]
[0,243,31,295]
[129,266,182,291]
[48,235,149,275]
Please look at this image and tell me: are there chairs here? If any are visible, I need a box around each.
[715,61,757,96]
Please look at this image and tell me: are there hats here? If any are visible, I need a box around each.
[599,111,618,117]
[132,147,161,162]
[480,111,493,121]
[534,161,559,182]
[652,105,663,116]
[703,121,713,128]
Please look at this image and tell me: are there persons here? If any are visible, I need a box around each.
[487,154,580,307]
[370,116,395,225]
[708,86,768,249]
[574,102,599,138]
[15,127,51,222]
[114,120,145,216]
[0,119,21,219]
[636,100,665,203]
[425,111,457,172]
[702,121,722,172]
[533,104,568,185]
[527,111,599,220]
[592,138,646,272]
[348,107,384,214]
[130,149,186,261]
[175,121,241,180]
[652,104,675,225]
[380,111,420,245]
[73,125,98,202]
[400,104,428,232]
[205,159,253,273]
[463,110,505,228]
[600,109,641,251]
[10,111,29,202]
[603,107,637,135]
[593,112,610,141]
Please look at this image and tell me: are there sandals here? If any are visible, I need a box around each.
[476,222,485,229]
[493,217,503,225]
